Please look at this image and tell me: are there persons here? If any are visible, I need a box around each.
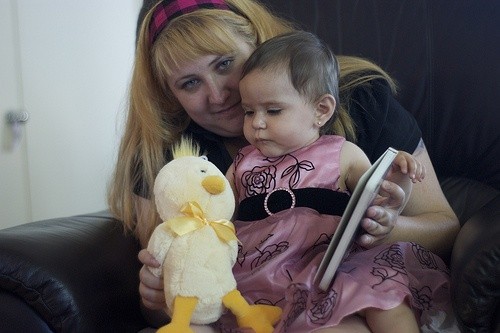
[222,29,454,332]
[106,0,463,333]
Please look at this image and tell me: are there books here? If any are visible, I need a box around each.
[311,147,399,291]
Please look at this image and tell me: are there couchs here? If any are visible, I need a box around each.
[1,2,499,331]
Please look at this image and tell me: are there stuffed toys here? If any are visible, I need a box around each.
[147,134,283,333]
